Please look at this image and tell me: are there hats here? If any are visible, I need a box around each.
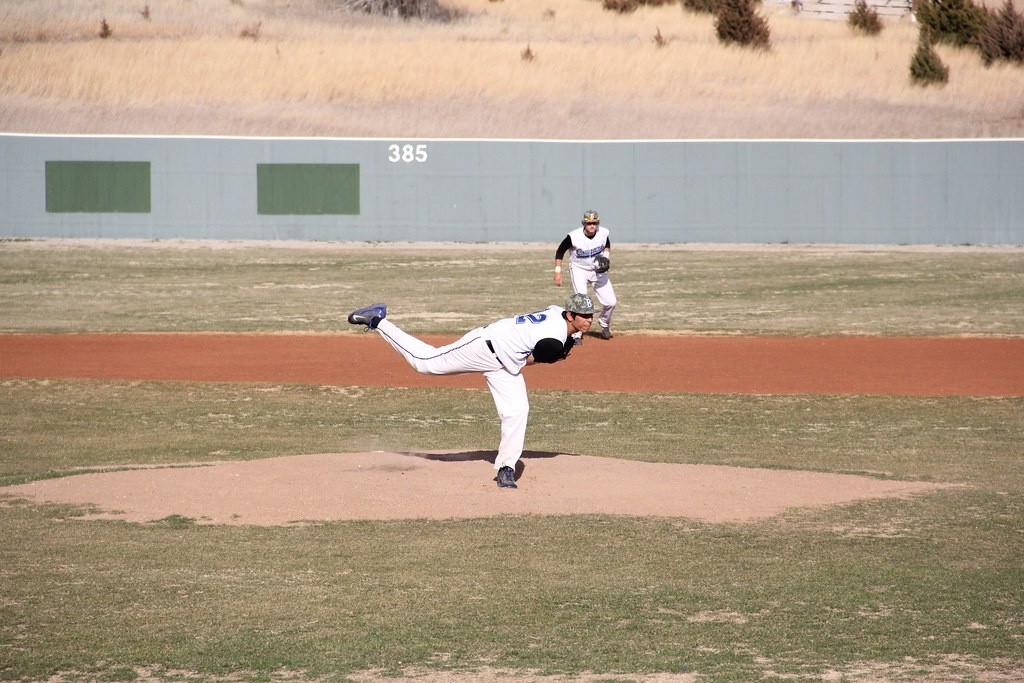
[565,292,601,313]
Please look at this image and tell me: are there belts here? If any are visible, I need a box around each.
[483,325,503,364]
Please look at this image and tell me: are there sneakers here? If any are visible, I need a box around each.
[348,304,386,332]
[599,322,611,337]
[574,337,583,344]
[497,462,517,488]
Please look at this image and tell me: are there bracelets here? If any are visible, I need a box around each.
[555,266,562,273]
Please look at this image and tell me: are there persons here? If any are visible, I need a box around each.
[351,294,599,488]
[555,209,617,340]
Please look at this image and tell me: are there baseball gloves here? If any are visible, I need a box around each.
[594,256,610,274]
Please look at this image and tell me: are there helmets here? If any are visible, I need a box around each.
[582,210,599,224]
